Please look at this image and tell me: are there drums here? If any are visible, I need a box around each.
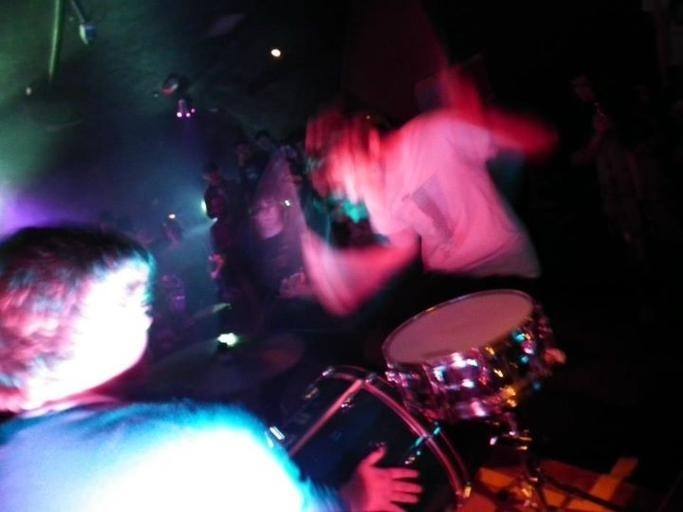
[382,290,554,420]
[265,365,471,511]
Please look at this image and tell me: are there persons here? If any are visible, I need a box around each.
[0,64,557,511]
